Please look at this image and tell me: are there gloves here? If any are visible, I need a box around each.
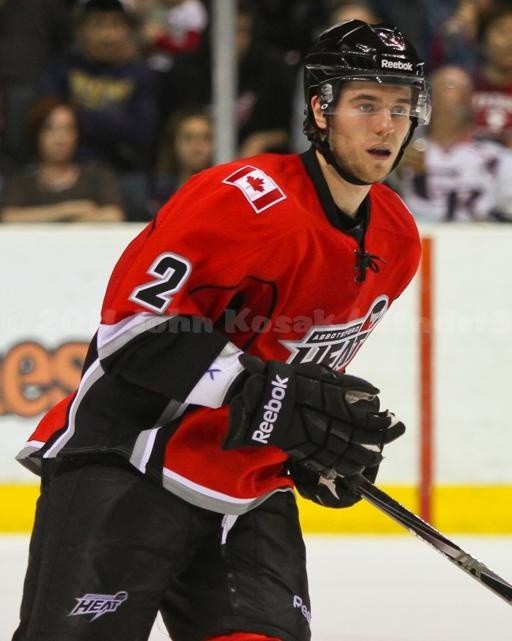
[220,351,392,480]
[287,453,378,506]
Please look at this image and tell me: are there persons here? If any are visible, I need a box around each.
[1,1,512,225]
[11,20,429,641]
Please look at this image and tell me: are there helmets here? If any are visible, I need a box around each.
[303,20,428,137]
